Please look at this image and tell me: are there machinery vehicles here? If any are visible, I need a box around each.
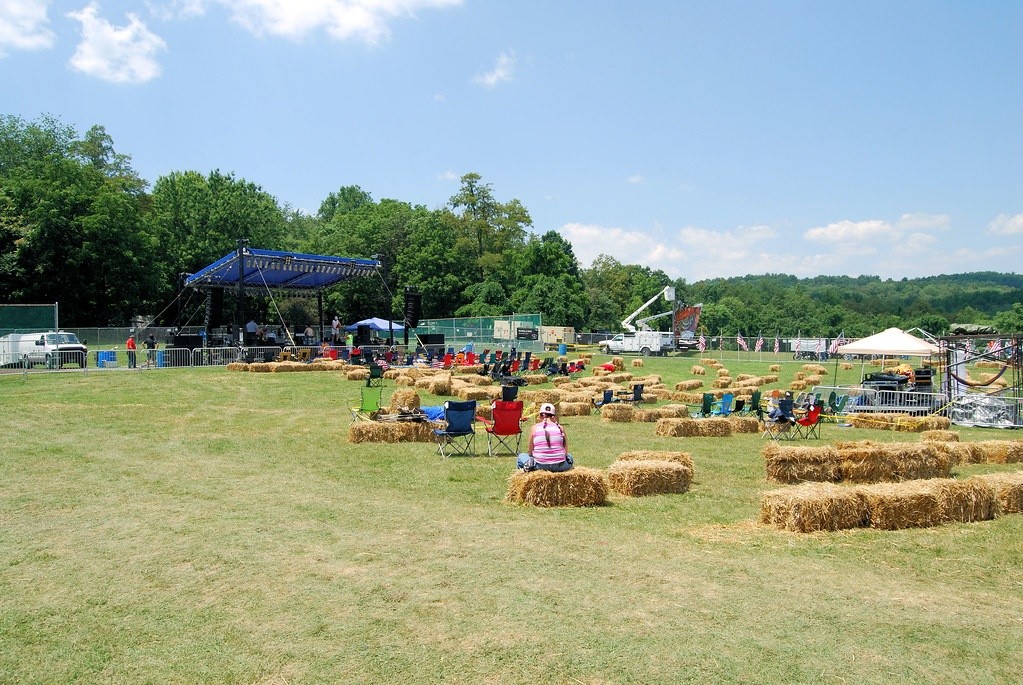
[636,303,703,353]
[598,286,663,359]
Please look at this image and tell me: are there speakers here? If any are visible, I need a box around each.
[357,325,370,344]
[403,293,422,327]
[204,285,224,328]
[272,339,285,346]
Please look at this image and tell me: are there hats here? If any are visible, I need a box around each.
[334,316,338,320]
[130,335,135,337]
[540,403,556,416]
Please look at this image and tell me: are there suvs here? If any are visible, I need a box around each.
[19,330,89,369]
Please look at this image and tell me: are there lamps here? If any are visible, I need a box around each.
[246,258,377,277]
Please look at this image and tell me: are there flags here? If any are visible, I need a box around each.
[793,337,801,352]
[830,333,841,354]
[754,332,763,352]
[991,339,1001,356]
[696,334,706,353]
[774,334,779,354]
[737,332,749,352]
[815,336,822,356]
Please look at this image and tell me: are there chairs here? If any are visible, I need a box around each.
[432,400,476,460]
[497,385,518,402]
[341,348,584,388]
[591,383,644,414]
[476,401,529,458]
[686,390,848,441]
[344,386,383,426]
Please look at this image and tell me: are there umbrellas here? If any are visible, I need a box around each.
[343,317,405,337]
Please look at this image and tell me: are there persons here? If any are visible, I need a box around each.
[255,327,268,337]
[126,335,137,368]
[304,324,314,338]
[142,334,159,367]
[331,316,341,342]
[227,321,233,341]
[245,319,258,344]
[502,362,511,376]
[278,323,290,339]
[517,403,573,472]
[344,330,353,359]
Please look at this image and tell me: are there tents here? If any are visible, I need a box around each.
[837,327,947,398]
